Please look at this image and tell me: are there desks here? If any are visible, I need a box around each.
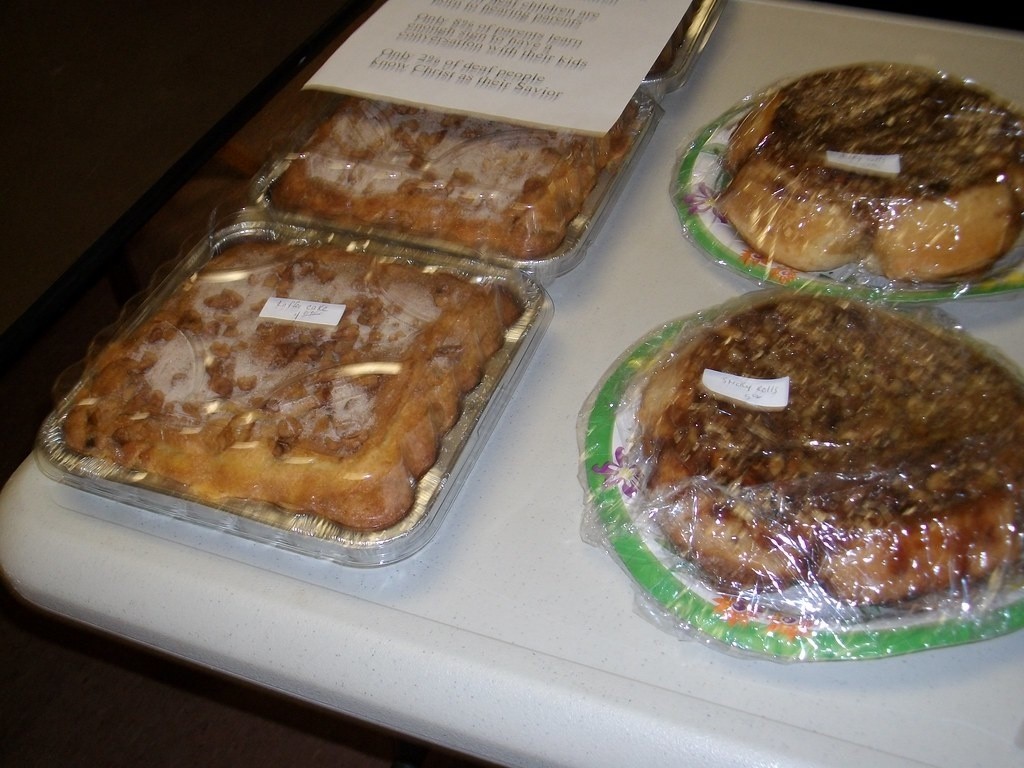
[0,0,1024,768]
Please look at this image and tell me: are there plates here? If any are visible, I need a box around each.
[584,310,1023,661]
[34,209,542,548]
[675,96,1024,303]
[250,92,656,272]
[639,0,726,92]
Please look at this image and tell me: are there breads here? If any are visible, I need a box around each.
[637,298,1024,606]
[64,242,524,529]
[267,96,639,258]
[716,62,1024,281]
